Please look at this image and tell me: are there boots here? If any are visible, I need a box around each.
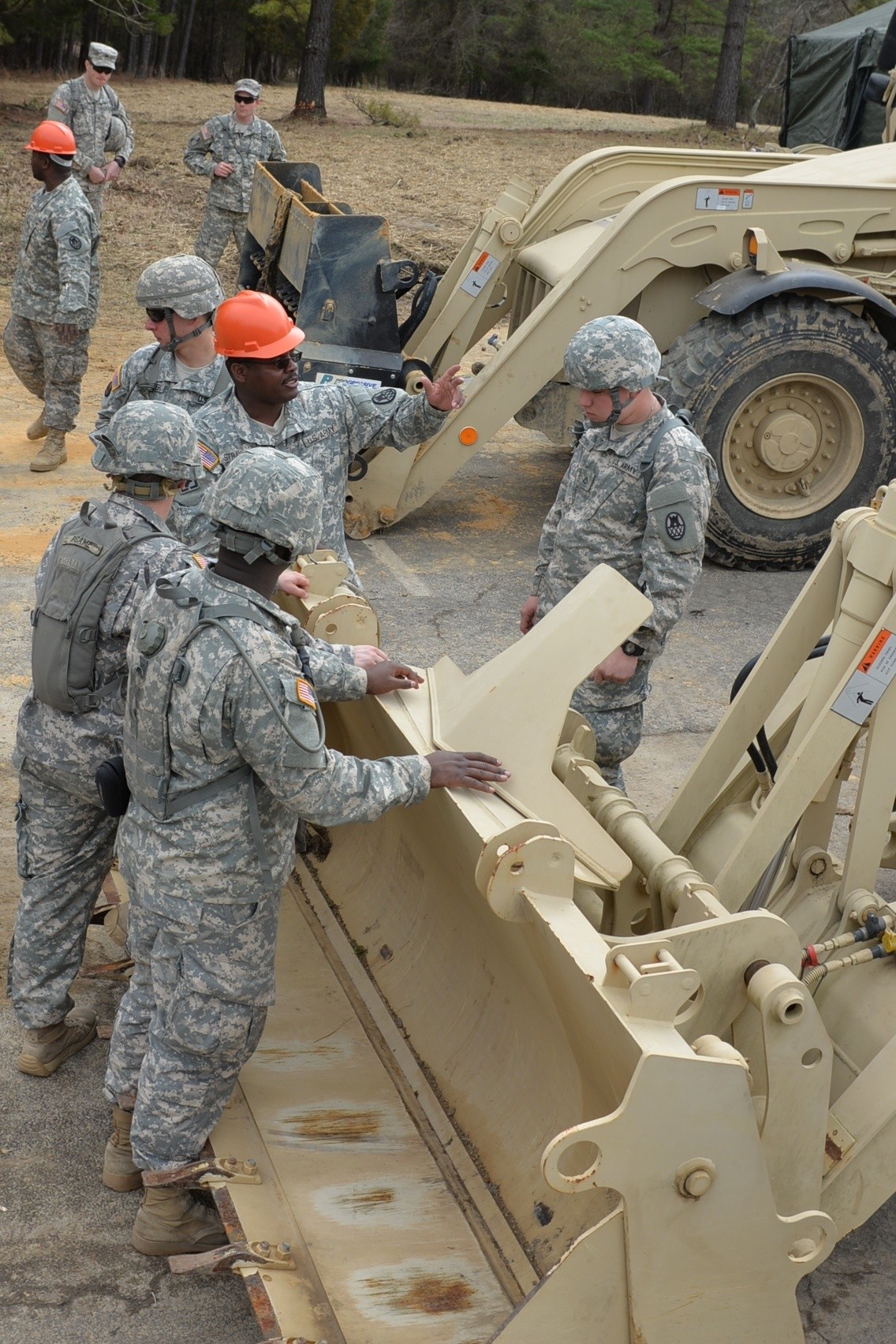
[19,1007,96,1077]
[30,426,67,471]
[103,1102,142,1192]
[27,408,50,439]
[133,1186,229,1255]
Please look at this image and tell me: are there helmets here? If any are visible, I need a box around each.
[213,290,305,358]
[23,120,77,155]
[90,400,206,481]
[104,116,126,152]
[199,447,323,552]
[564,315,662,391]
[136,253,225,319]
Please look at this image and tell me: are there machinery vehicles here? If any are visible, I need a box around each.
[222,73,896,575]
[102,468,895,1343]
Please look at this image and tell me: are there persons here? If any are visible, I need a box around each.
[2,121,100,472]
[104,290,467,947]
[7,400,388,1083]
[88,254,226,481]
[182,78,288,273]
[47,42,135,223]
[518,314,719,797]
[101,452,511,1257]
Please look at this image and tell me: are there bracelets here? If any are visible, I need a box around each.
[114,155,126,168]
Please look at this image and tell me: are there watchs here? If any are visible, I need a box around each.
[621,640,645,657]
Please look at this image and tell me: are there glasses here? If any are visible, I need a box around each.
[93,66,112,74]
[146,308,174,322]
[239,348,302,368]
[234,94,255,104]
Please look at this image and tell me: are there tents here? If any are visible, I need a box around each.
[778,0,896,151]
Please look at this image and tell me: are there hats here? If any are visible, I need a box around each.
[88,42,118,70]
[234,78,262,98]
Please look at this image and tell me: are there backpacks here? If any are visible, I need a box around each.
[29,498,179,715]
